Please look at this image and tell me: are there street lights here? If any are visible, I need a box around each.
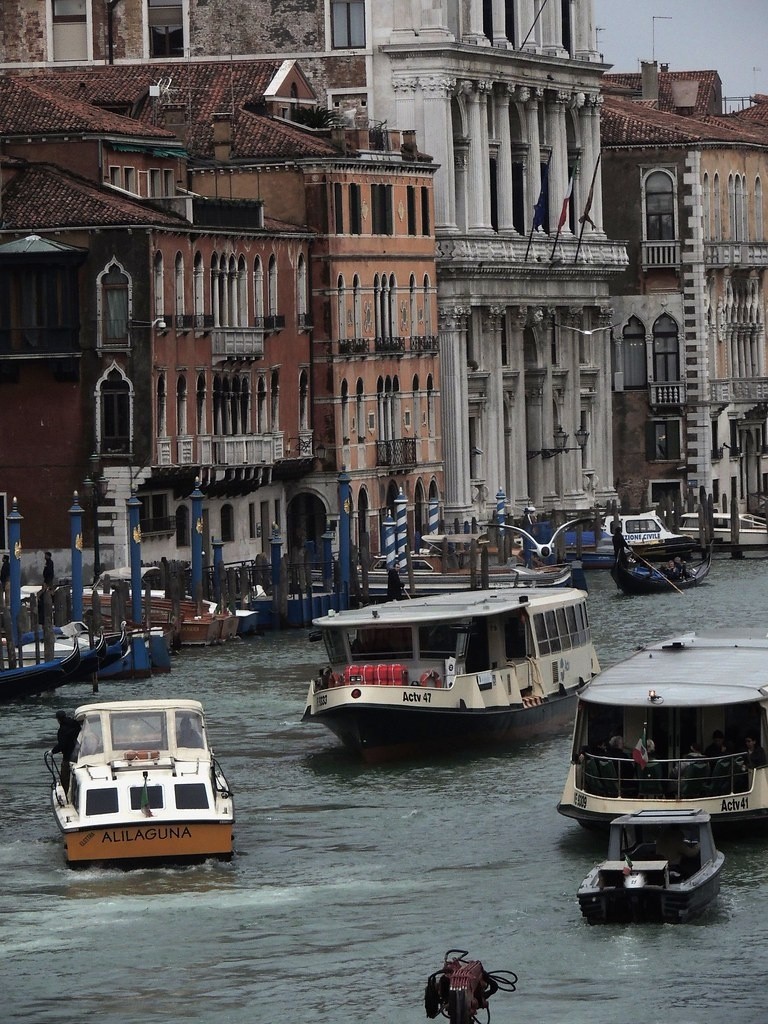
[82,451,109,581]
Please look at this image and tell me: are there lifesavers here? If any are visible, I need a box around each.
[420,670,442,688]
[328,672,339,688]
[124,750,160,760]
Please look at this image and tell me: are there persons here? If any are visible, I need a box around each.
[43,552,54,584]
[0,554,10,586]
[612,527,628,564]
[48,711,81,758]
[578,729,765,776]
[667,829,701,871]
[665,556,684,578]
[388,563,404,600]
[177,718,201,748]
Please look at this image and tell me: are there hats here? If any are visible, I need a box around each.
[713,730,724,739]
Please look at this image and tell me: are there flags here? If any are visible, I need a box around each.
[579,153,600,229]
[533,151,553,234]
[558,154,577,231]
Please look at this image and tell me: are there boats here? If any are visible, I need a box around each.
[523,521,632,570]
[299,588,601,766]
[557,627,768,830]
[602,510,693,544]
[611,548,714,596]
[50,699,236,871]
[576,809,728,925]
[0,567,259,700]
[678,512,768,544]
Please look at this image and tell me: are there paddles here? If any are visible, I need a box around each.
[627,548,684,595]
